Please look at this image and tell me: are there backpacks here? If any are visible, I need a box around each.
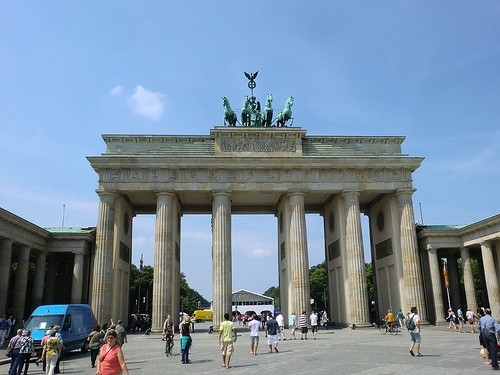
[405,314,416,330]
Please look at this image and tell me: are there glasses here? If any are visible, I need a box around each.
[107,337,114,339]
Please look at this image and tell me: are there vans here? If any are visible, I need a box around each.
[193,310,213,323]
[21,304,94,360]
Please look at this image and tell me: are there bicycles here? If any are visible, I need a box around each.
[379,319,398,335]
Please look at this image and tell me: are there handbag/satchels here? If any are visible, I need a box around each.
[6,349,12,357]
[469,318,474,324]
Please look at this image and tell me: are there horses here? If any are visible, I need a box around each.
[241,95,250,126]
[222,95,237,126]
[276,96,294,127]
[261,95,273,126]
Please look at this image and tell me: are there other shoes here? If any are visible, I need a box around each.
[36,361,39,366]
[182,361,185,364]
[92,365,95,368]
[409,350,416,356]
[269,351,272,353]
[226,366,229,368]
[492,364,499,369]
[274,347,278,353]
[254,353,257,355]
[186,361,189,363]
[416,353,424,356]
[222,364,226,367]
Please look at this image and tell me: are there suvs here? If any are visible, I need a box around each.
[131,314,150,323]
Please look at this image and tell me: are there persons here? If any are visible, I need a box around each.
[395,309,403,327]
[87,320,128,368]
[276,312,286,341]
[409,307,423,357]
[309,311,318,340]
[457,306,464,333]
[299,311,308,339]
[0,314,65,375]
[248,314,261,355]
[236,312,265,330]
[162,315,174,356]
[218,313,237,368]
[446,309,458,330]
[289,312,298,340]
[265,315,280,353]
[128,316,152,334]
[384,309,395,332]
[466,308,475,333]
[178,315,193,364]
[477,306,500,370]
[97,330,129,375]
